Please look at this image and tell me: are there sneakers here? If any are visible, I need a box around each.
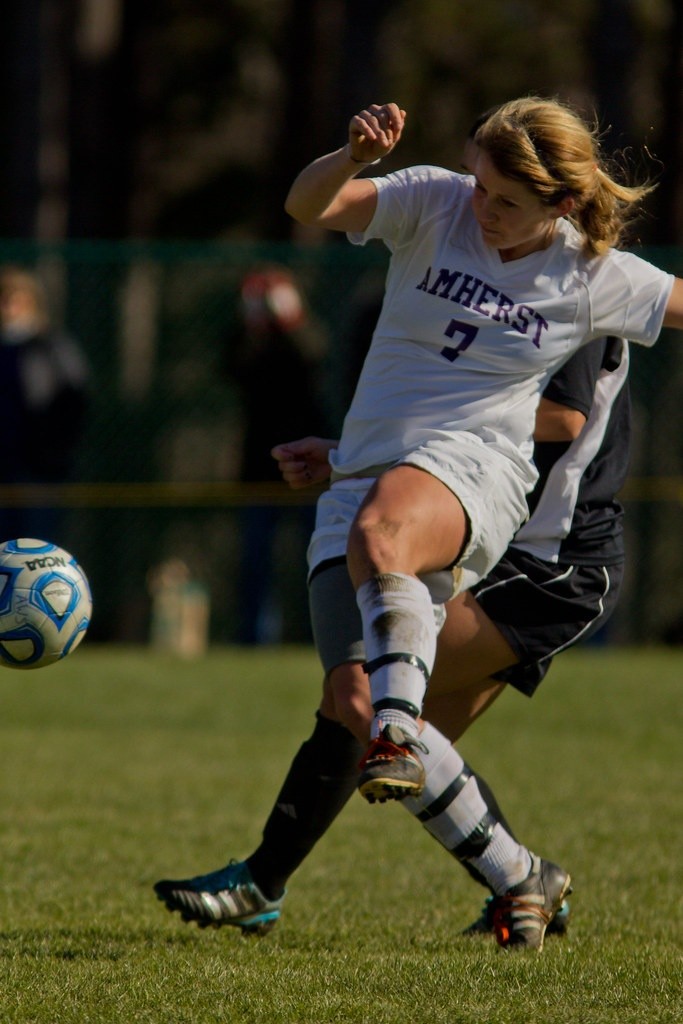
[463,887,573,935]
[153,857,287,937]
[355,719,429,804]
[491,850,571,952]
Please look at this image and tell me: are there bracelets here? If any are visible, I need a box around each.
[346,142,381,165]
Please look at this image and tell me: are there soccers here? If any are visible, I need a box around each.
[0,538,95,671]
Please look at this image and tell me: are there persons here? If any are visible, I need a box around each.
[221,266,342,649]
[284,98,682,950]
[151,335,631,941]
[0,261,113,546]
